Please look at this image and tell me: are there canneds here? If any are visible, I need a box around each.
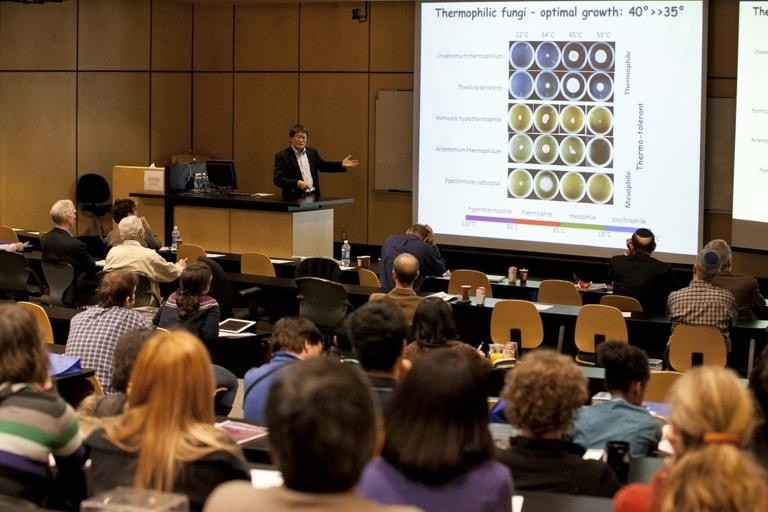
[502,345,514,360]
[476,287,486,304]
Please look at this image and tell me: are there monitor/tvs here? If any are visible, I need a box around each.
[205,161,237,192]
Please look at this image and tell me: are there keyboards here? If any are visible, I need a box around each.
[223,191,252,197]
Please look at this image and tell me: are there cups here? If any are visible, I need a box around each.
[606,441,631,484]
[489,344,503,354]
[459,286,472,301]
[477,285,485,306]
[24,245,33,253]
[520,269,527,283]
[358,256,370,269]
[508,341,517,357]
[174,238,183,248]
[505,345,515,359]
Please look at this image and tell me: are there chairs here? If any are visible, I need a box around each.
[75,172,113,241]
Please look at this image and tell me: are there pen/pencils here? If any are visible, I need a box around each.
[477,341,484,351]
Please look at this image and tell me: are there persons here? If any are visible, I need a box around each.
[1,300,94,511]
[343,299,412,420]
[399,296,492,377]
[379,224,446,292]
[152,263,238,417]
[488,396,524,451]
[243,314,325,428]
[274,124,359,197]
[613,365,768,512]
[87,327,251,511]
[704,239,766,322]
[74,327,163,419]
[332,317,353,360]
[0,242,24,253]
[748,342,768,472]
[494,346,633,499]
[64,269,154,392]
[102,214,189,307]
[40,199,99,309]
[358,343,514,512]
[369,250,426,344]
[567,340,661,460]
[200,354,427,512]
[662,249,739,373]
[106,199,161,251]
[604,228,676,318]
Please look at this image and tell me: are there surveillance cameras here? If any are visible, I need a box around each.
[351,8,361,17]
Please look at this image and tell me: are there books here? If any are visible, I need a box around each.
[216,419,268,444]
[658,423,676,455]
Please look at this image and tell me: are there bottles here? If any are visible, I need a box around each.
[171,226,180,249]
[198,173,202,190]
[202,172,207,189]
[194,173,200,192]
[341,239,350,266]
